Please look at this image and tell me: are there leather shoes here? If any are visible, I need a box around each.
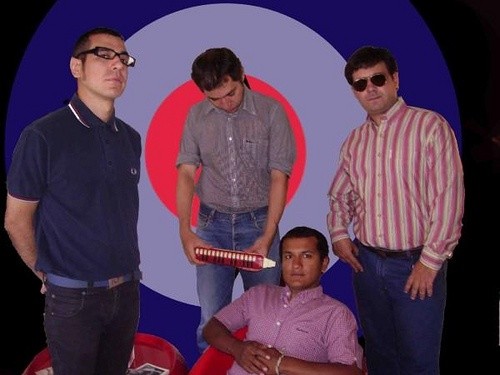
[356,238,422,257]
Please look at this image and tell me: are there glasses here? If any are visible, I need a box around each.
[349,74,387,92]
[75,45,136,67]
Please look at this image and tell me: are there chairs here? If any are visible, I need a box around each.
[19,331,183,375]
[185,327,368,374]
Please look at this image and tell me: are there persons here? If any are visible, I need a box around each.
[202,226,366,375]
[326,45,465,375]
[176,47,296,355]
[4,27,143,375]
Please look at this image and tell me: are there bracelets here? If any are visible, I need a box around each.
[275,355,285,375]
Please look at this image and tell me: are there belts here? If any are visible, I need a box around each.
[44,271,143,290]
